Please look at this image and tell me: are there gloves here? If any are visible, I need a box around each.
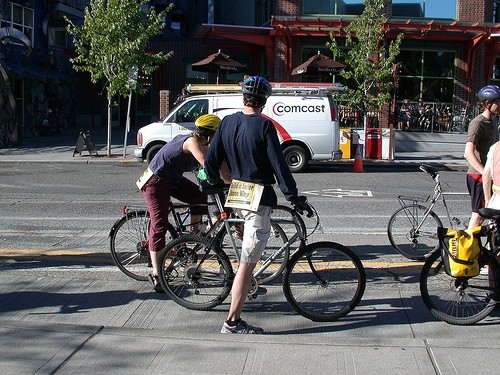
[203,177,225,189]
[289,195,313,217]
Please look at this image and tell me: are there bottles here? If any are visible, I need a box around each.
[197,220,208,236]
[459,219,469,230]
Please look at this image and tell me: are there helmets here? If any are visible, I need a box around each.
[195,113,221,129]
[242,76,273,99]
[477,85,500,102]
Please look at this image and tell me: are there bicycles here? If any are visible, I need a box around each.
[156,182,367,322]
[387,165,500,261]
[438,106,454,134]
[419,208,500,325]
[108,187,291,285]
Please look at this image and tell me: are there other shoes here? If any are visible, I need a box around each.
[255,286,267,295]
[220,318,263,334]
[148,273,162,293]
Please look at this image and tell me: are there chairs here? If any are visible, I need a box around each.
[336,103,484,132]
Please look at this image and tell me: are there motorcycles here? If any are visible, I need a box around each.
[396,99,417,131]
[417,101,432,131]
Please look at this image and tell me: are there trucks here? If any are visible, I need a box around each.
[133,94,343,173]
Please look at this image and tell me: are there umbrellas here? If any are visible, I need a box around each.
[292,51,346,82]
[192,48,248,84]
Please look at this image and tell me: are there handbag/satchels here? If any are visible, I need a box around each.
[435,226,485,277]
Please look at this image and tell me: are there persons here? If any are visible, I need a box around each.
[400,99,425,129]
[464,85,500,229]
[139,113,245,300]
[203,74,313,334]
[482,140,500,302]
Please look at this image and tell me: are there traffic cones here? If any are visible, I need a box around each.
[351,145,365,173]
